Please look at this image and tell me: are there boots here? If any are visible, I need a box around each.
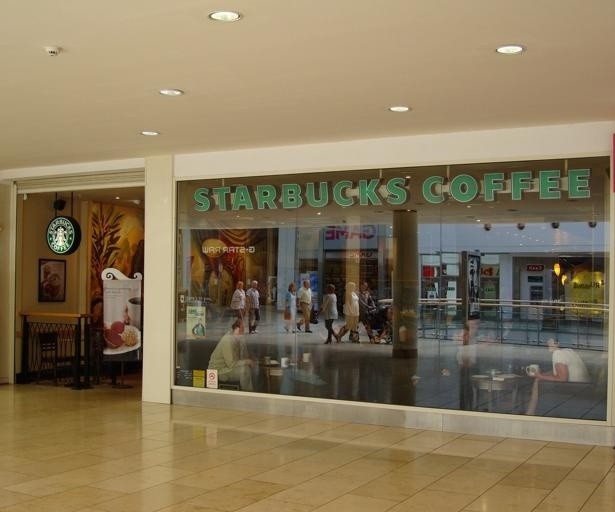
[351,331,360,343]
[335,326,348,343]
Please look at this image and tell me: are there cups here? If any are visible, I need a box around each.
[128,297,142,331]
[264,356,271,366]
[303,352,310,362]
[281,357,288,369]
[526,364,539,377]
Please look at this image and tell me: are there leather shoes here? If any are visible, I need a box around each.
[297,322,301,330]
[304,330,312,333]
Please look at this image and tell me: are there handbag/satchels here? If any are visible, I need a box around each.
[231,301,239,309]
[309,310,319,324]
[283,311,292,320]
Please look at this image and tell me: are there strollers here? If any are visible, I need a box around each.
[366,304,394,344]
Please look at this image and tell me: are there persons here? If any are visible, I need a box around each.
[230,281,246,329]
[283,283,299,333]
[296,280,312,333]
[245,281,261,333]
[336,280,376,342]
[281,329,335,395]
[316,283,341,344]
[342,282,360,342]
[206,319,261,391]
[524,331,590,416]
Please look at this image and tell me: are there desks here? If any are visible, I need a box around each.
[177,341,608,422]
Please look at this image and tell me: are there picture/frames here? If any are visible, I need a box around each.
[38,258,66,302]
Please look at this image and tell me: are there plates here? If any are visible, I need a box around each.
[102,325,141,356]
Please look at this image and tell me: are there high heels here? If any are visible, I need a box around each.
[324,337,333,344]
